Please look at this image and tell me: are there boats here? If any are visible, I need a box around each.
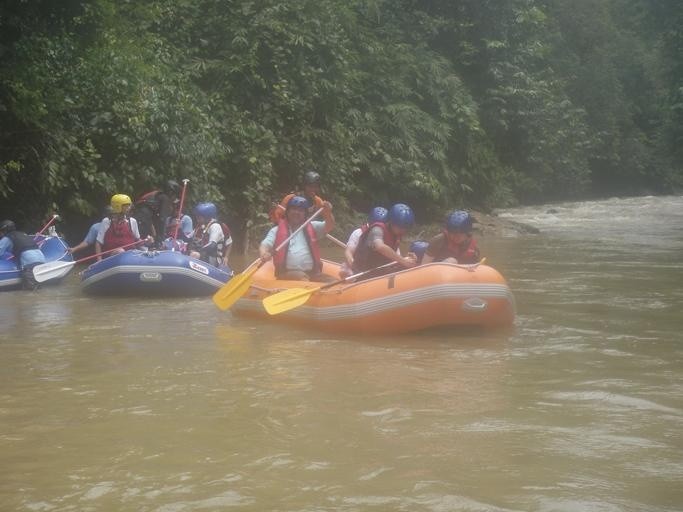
[0,234,73,290]
[79,249,233,295]
[230,257,517,332]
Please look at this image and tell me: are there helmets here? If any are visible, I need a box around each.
[386,202,415,228]
[302,171,321,184]
[205,202,216,216]
[447,210,473,233]
[193,203,212,223]
[111,194,132,213]
[0,219,16,232]
[409,240,429,258]
[286,196,308,211]
[173,198,179,207]
[163,179,180,194]
[368,206,390,224]
[106,205,114,215]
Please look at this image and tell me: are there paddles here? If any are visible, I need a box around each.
[263,260,398,316]
[33,239,145,282]
[213,206,325,311]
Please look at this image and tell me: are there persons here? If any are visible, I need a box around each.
[340,206,400,278]
[259,197,335,280]
[271,172,323,222]
[217,220,232,266]
[95,193,148,260]
[355,204,418,269]
[421,210,481,265]
[186,203,223,267]
[65,205,115,256]
[165,203,192,254]
[0,220,45,291]
[128,190,171,250]
